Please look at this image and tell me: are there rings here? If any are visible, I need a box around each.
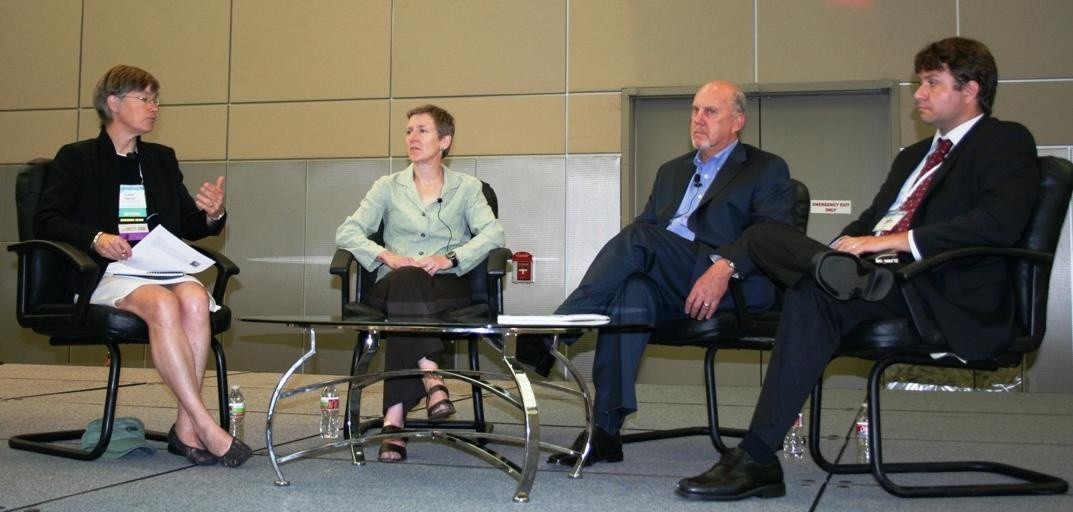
[209,200,214,208]
[703,302,711,308]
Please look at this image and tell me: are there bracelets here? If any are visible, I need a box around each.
[90,230,104,249]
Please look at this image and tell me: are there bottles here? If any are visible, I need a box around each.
[320,385,341,440]
[784,408,803,460]
[227,384,245,442]
[857,401,871,464]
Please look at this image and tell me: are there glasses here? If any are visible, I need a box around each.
[119,94,160,106]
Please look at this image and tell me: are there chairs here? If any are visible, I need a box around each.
[330,180,512,445]
[599,179,809,459]
[8,158,240,461]
[810,155,1073,498]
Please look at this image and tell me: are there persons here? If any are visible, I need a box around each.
[675,35,1042,503]
[333,104,507,464]
[31,64,253,468]
[515,79,796,470]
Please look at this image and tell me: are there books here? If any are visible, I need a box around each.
[496,313,611,325]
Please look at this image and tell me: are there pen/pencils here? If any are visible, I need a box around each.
[122,235,130,256]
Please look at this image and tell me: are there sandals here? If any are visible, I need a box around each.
[211,436,253,467]
[426,385,456,422]
[378,426,408,463]
[166,424,217,466]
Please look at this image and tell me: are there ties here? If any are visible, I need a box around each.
[881,137,953,238]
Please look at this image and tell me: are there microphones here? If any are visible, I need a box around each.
[437,198,452,252]
[672,174,703,219]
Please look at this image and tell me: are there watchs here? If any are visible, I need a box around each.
[727,259,737,269]
[445,250,459,268]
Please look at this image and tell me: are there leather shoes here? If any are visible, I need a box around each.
[547,427,624,468]
[812,249,894,301]
[674,447,786,501]
[516,334,555,377]
[887,262,916,268]
